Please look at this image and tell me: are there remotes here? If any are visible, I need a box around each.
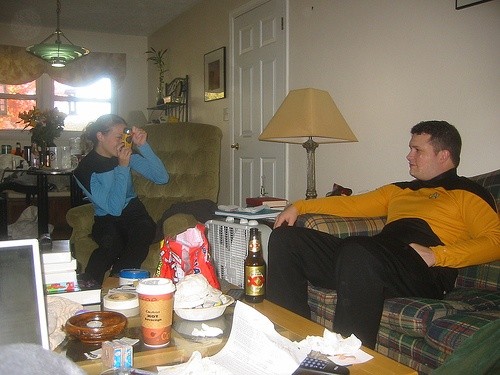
[299,357,350,375]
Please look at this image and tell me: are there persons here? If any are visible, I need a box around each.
[70,114,169,290]
[265,120,500,350]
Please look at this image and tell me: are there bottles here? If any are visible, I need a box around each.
[30,143,40,169]
[244,228,266,303]
[15,142,23,169]
[119,269,149,286]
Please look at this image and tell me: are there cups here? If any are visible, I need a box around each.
[50,146,71,170]
[2,145,11,154]
[135,278,176,348]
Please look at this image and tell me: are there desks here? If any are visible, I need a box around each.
[45,277,417,375]
[0,152,79,240]
[217,207,290,230]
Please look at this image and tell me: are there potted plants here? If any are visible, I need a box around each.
[145,46,172,104]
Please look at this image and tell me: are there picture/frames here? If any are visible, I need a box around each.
[203,46,225,102]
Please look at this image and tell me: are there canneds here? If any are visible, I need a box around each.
[2,145,11,154]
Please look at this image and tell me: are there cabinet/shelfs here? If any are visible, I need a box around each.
[147,75,189,122]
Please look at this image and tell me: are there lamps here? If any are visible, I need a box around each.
[25,0,90,67]
[258,88,359,199]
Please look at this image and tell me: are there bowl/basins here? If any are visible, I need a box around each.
[173,294,235,321]
[66,311,127,343]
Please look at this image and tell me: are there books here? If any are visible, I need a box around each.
[215,196,288,221]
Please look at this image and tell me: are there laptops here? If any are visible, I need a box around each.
[0,238,51,351]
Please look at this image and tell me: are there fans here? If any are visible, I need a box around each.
[204,216,273,290]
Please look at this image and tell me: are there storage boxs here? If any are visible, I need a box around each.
[38,240,79,291]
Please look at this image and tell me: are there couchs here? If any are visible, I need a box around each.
[294,169,500,375]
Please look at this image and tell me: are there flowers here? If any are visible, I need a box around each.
[17,106,68,146]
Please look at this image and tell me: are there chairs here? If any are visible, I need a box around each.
[65,122,225,286]
